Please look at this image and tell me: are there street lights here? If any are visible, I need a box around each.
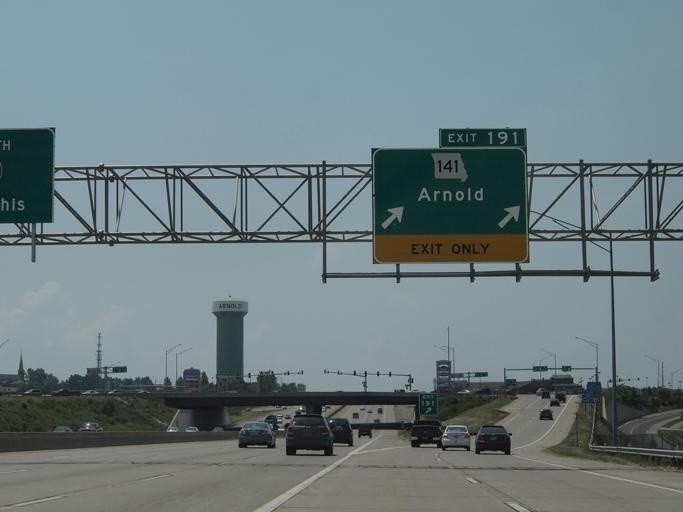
[538,348,557,379]
[432,344,454,381]
[576,336,598,370]
[164,342,192,385]
[644,354,664,388]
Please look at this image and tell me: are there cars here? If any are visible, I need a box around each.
[239,405,383,456]
[79,423,103,433]
[53,426,73,432]
[184,427,199,432]
[24,389,149,396]
[539,409,552,420]
[411,420,512,454]
[212,426,224,432]
[167,427,179,432]
[536,388,566,406]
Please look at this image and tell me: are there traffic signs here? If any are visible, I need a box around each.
[371,148,529,263]
[419,393,438,417]
